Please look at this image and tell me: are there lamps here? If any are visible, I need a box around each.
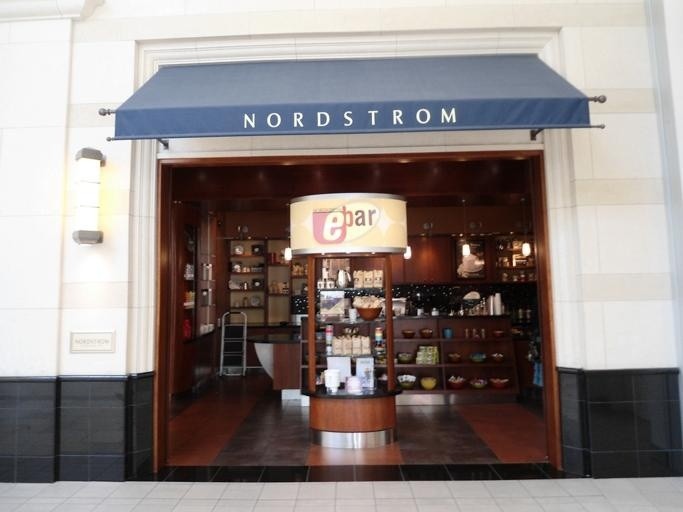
[71,148,105,245]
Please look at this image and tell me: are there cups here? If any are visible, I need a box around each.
[349,309,357,321]
[417,308,424,316]
[444,328,453,337]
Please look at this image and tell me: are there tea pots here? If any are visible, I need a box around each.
[338,269,352,287]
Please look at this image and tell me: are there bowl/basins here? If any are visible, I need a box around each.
[402,328,433,339]
[398,375,436,390]
[356,307,382,321]
[447,352,509,389]
[398,353,414,363]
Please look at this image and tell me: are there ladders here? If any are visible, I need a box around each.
[219,311,247,376]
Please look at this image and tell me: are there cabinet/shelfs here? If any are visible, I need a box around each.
[282,246,406,448]
[450,234,537,281]
[296,312,544,405]
[168,197,219,412]
[220,234,310,391]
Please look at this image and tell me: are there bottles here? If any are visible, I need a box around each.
[479,298,487,315]
[317,277,335,290]
[517,307,534,343]
[243,280,248,307]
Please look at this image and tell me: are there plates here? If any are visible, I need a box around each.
[233,245,245,256]
[250,296,262,307]
